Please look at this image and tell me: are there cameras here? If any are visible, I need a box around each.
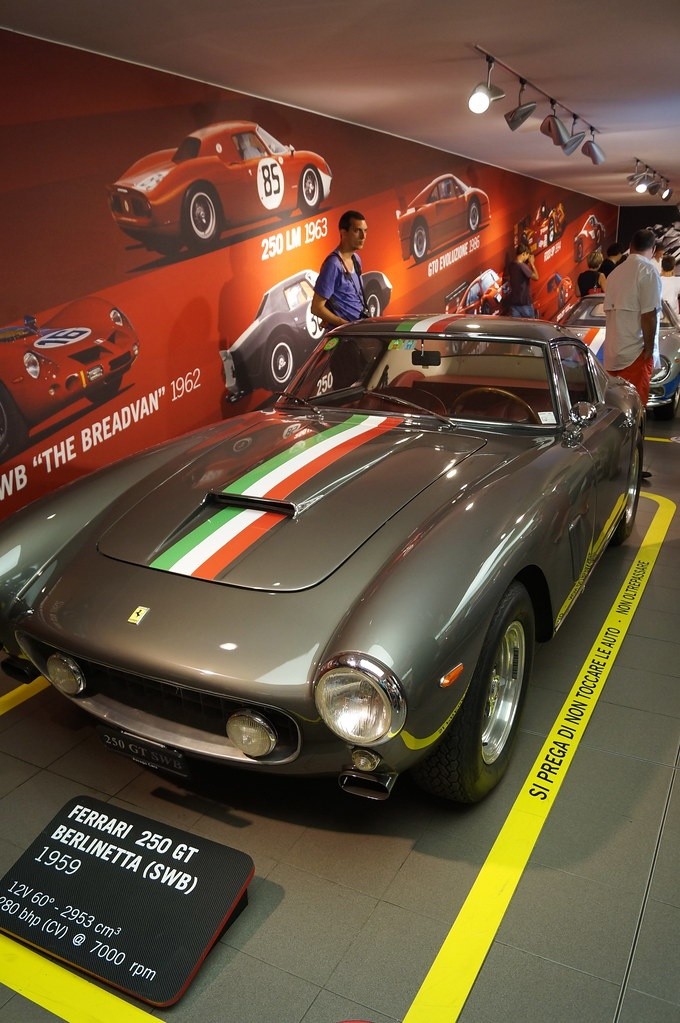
[360,308,371,319]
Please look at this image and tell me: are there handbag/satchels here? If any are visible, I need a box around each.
[589,272,601,295]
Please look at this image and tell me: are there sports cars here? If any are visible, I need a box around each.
[395,173,492,265]
[445,268,515,319]
[218,267,394,404]
[549,293,680,422]
[513,202,569,258]
[0,297,141,464]
[106,120,336,260]
[533,271,581,326]
[574,214,607,265]
[0,305,648,817]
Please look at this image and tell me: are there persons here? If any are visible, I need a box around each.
[603,230,662,406]
[598,246,622,280]
[650,244,680,315]
[310,211,367,391]
[576,252,606,297]
[510,244,539,319]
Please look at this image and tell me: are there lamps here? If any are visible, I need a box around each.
[626,160,647,187]
[562,114,585,155]
[581,127,607,166]
[657,176,670,199]
[504,78,536,131]
[469,56,507,115]
[664,181,672,202]
[636,167,656,193]
[648,172,660,195]
[539,98,571,145]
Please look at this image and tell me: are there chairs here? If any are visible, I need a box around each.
[359,387,447,416]
[498,388,553,423]
